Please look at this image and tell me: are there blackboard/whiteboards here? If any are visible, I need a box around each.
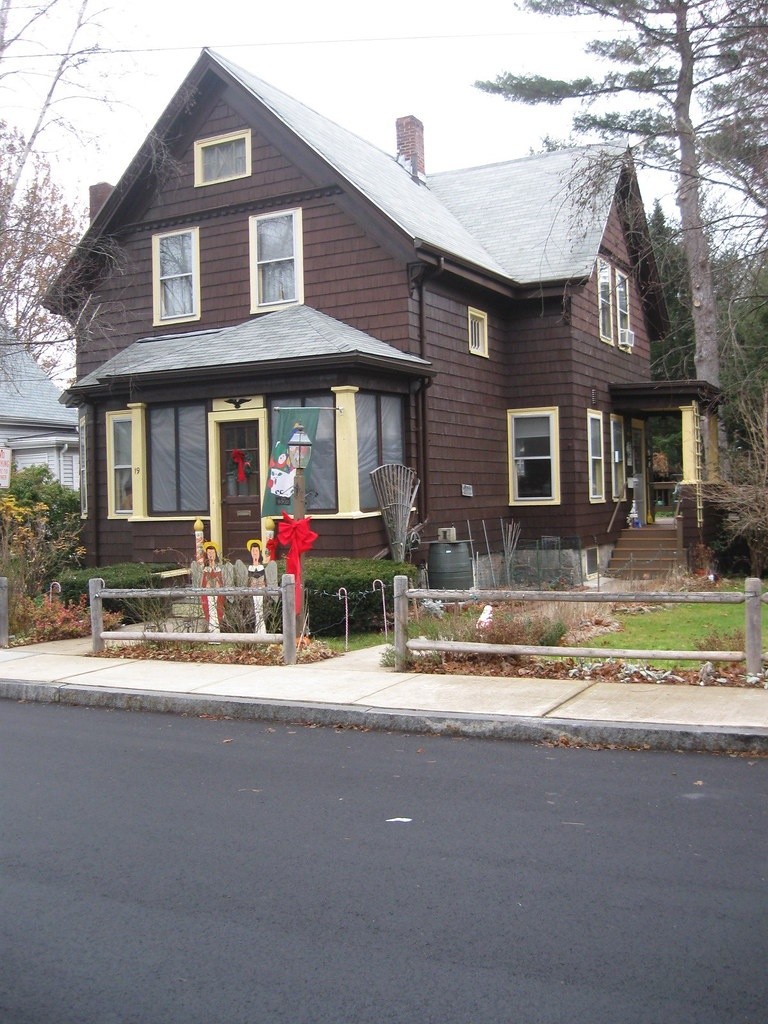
[0,447,12,489]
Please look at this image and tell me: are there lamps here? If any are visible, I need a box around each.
[287,425,313,469]
